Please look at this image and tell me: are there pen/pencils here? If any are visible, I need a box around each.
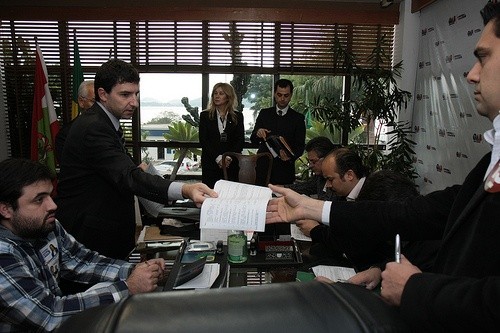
[144,261,152,266]
[156,252,160,265]
[395,234,401,264]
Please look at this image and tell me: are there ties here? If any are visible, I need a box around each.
[118,125,126,151]
[277,109,283,120]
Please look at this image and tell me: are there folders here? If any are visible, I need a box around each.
[265,136,295,160]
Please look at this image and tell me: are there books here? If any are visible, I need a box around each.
[312,265,360,285]
[291,224,312,241]
[199,180,273,232]
[136,226,184,247]
[259,134,295,160]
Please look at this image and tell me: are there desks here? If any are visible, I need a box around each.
[131,217,305,290]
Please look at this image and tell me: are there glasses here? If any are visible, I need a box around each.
[305,156,321,164]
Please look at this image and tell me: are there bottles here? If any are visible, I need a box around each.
[228,229,248,263]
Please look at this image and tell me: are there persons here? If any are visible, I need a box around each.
[54,59,217,260]
[250,79,306,187]
[0,158,165,333]
[199,83,244,189]
[284,136,421,254]
[266,1,500,333]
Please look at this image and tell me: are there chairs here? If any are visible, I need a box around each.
[222,152,273,187]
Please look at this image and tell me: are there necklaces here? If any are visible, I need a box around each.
[217,109,227,131]
